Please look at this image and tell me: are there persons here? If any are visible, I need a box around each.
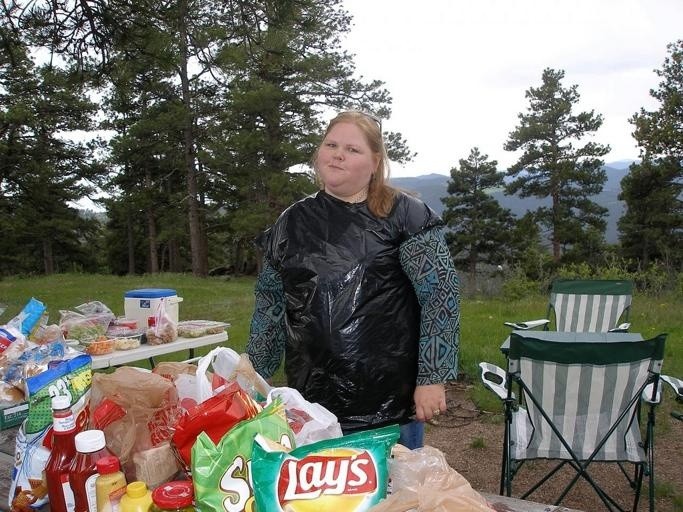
[244,111,461,450]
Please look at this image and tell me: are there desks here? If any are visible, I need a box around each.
[499,331,644,401]
[91,329,230,370]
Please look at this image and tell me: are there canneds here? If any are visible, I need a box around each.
[147,481,199,512]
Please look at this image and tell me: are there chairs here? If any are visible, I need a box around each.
[478,331,682,510]
[504,281,633,334]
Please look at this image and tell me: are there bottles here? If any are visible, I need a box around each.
[46,396,195,512]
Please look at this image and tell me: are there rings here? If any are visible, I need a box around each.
[434,410,441,416]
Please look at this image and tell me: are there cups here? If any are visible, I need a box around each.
[0,328,15,354]
